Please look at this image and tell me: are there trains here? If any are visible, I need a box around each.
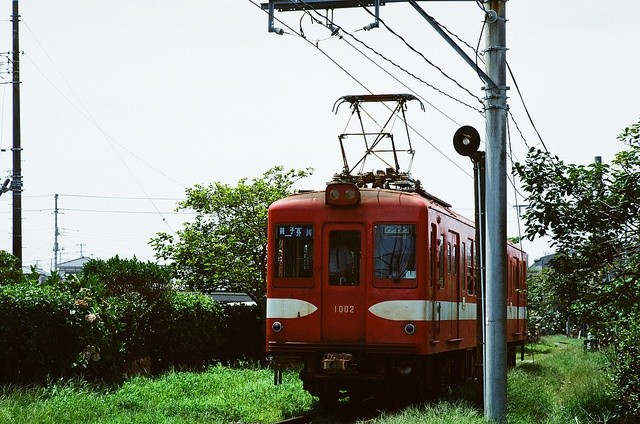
[267,92,527,407]
